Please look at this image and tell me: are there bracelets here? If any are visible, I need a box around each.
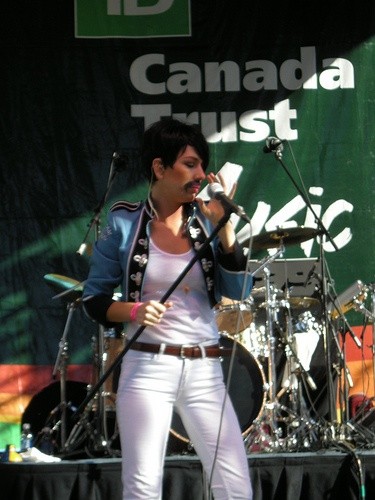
[129,302,143,321]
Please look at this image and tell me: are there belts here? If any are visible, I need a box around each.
[124,338,238,358]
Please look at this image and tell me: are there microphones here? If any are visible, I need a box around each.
[262,137,288,155]
[208,182,250,223]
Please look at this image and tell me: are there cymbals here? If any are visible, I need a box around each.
[279,297,320,316]
[43,274,85,304]
[242,227,318,249]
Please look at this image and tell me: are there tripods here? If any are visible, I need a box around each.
[32,141,375,459]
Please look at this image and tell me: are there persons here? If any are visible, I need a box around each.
[81,118,254,500]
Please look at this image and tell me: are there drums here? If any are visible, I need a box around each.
[85,328,128,413]
[230,320,271,359]
[213,295,253,334]
[170,331,269,446]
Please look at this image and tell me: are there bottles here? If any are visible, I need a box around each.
[18,423,33,462]
